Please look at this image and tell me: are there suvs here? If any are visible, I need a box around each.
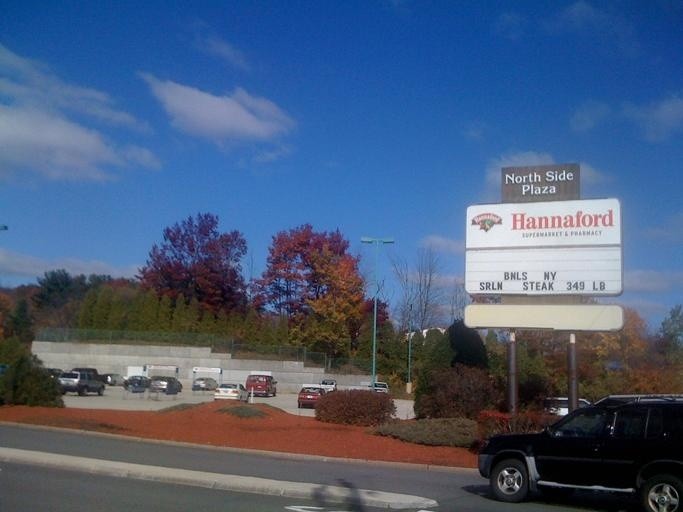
[475,393,682,512]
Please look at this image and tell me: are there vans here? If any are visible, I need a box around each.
[544,396,593,419]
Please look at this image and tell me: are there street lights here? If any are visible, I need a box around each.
[359,236,395,390]
[405,293,414,395]
[0,225,8,231]
